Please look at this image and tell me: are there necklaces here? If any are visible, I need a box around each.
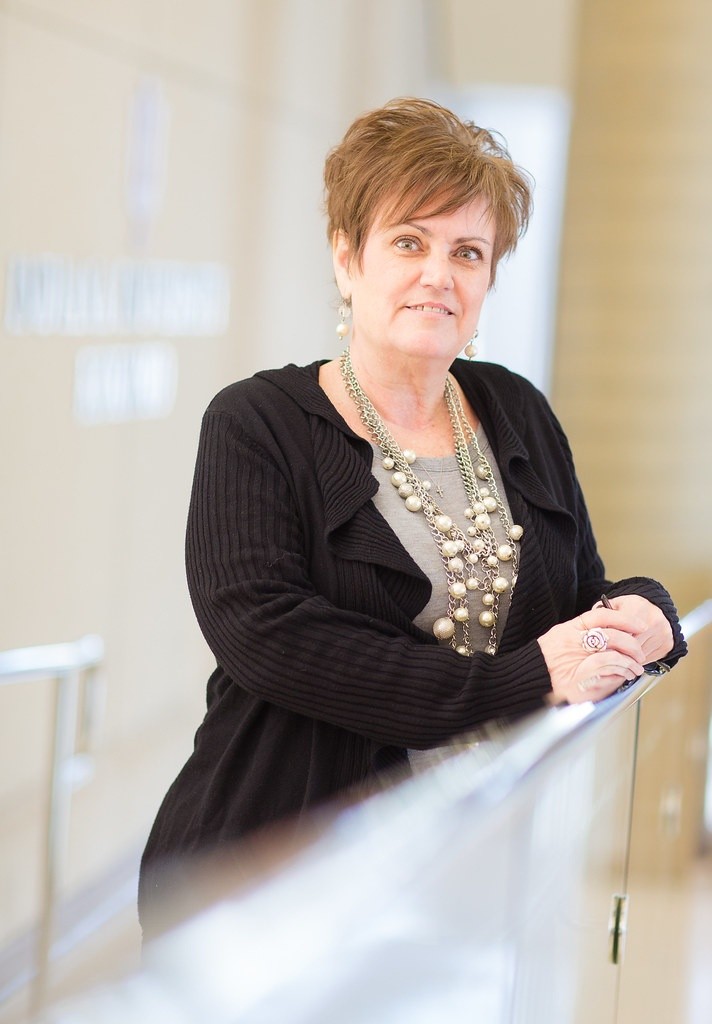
[341,344,522,658]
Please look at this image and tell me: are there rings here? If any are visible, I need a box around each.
[580,614,609,654]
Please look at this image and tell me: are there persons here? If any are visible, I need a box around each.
[137,98,689,941]
